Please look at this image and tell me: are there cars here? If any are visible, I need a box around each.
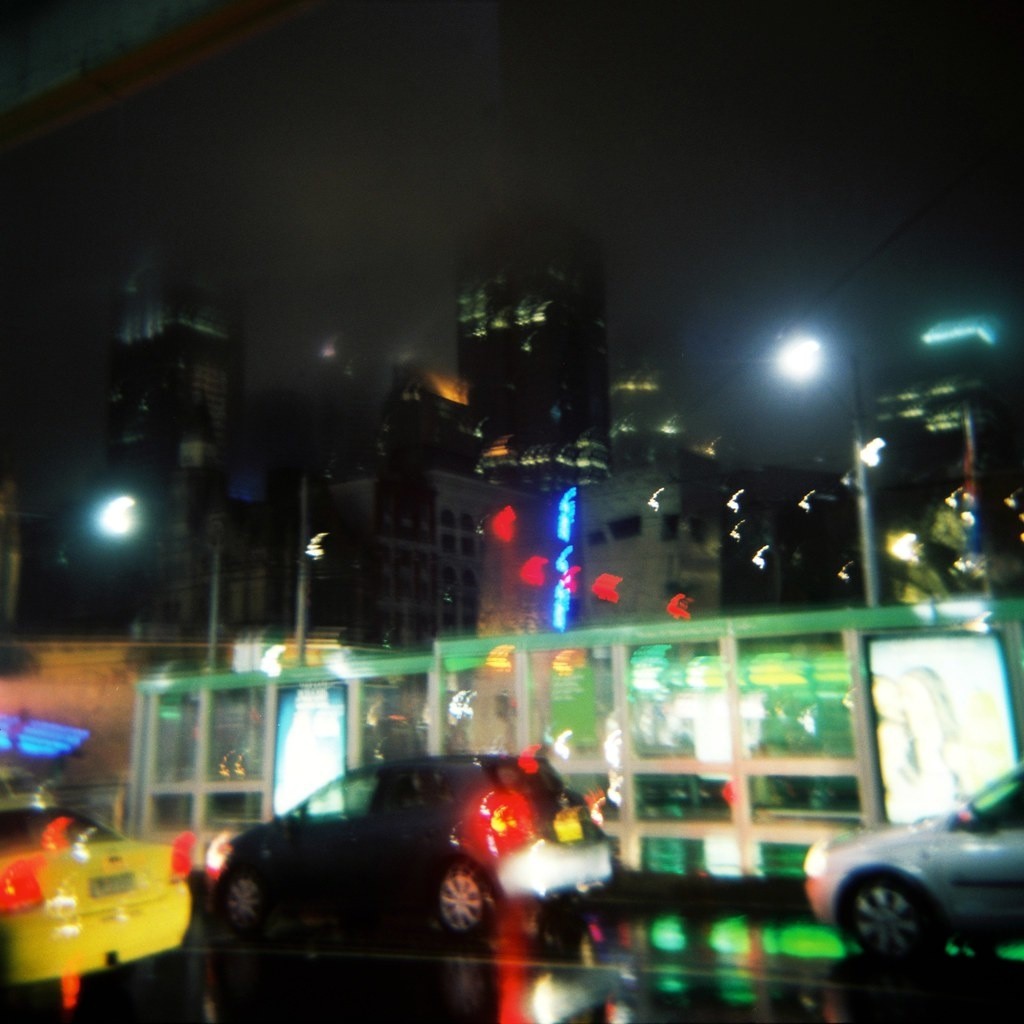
[801,764,1024,969]
[1,766,192,1010]
[208,755,616,947]
